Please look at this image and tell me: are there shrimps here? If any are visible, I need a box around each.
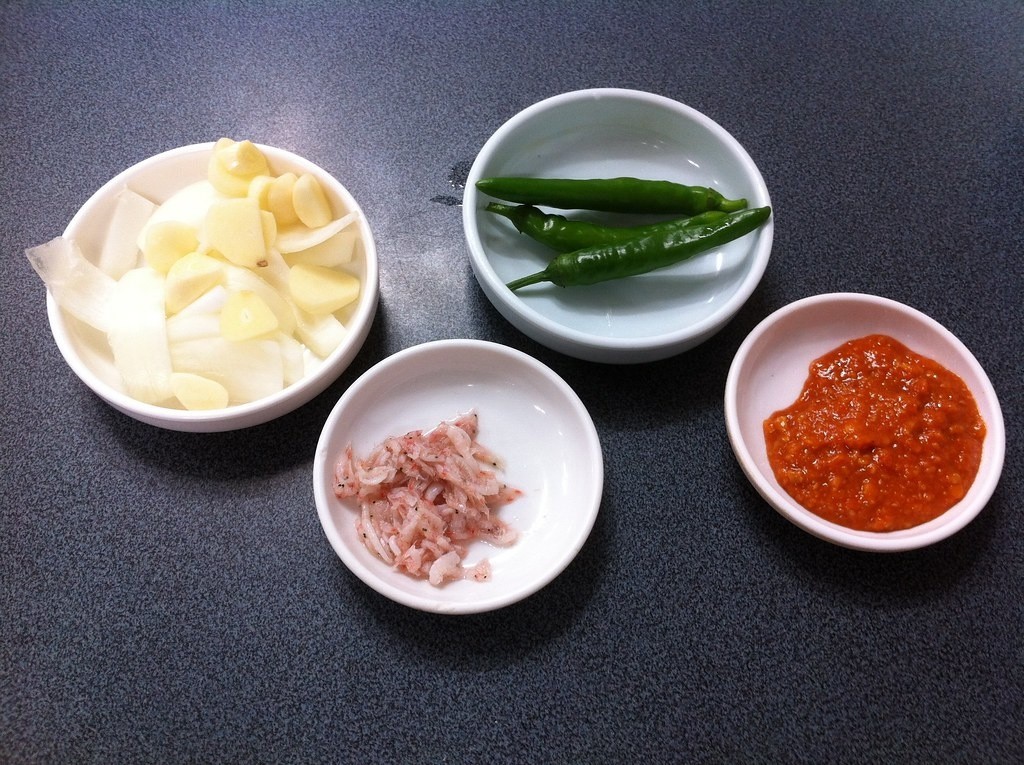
[331,402,526,586]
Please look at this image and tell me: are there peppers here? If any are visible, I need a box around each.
[475,176,772,291]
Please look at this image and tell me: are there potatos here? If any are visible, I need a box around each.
[144,137,360,414]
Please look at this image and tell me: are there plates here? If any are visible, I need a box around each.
[462,87,774,364]
[46,142,379,432]
[722,291,1006,551]
[312,337,604,614]
